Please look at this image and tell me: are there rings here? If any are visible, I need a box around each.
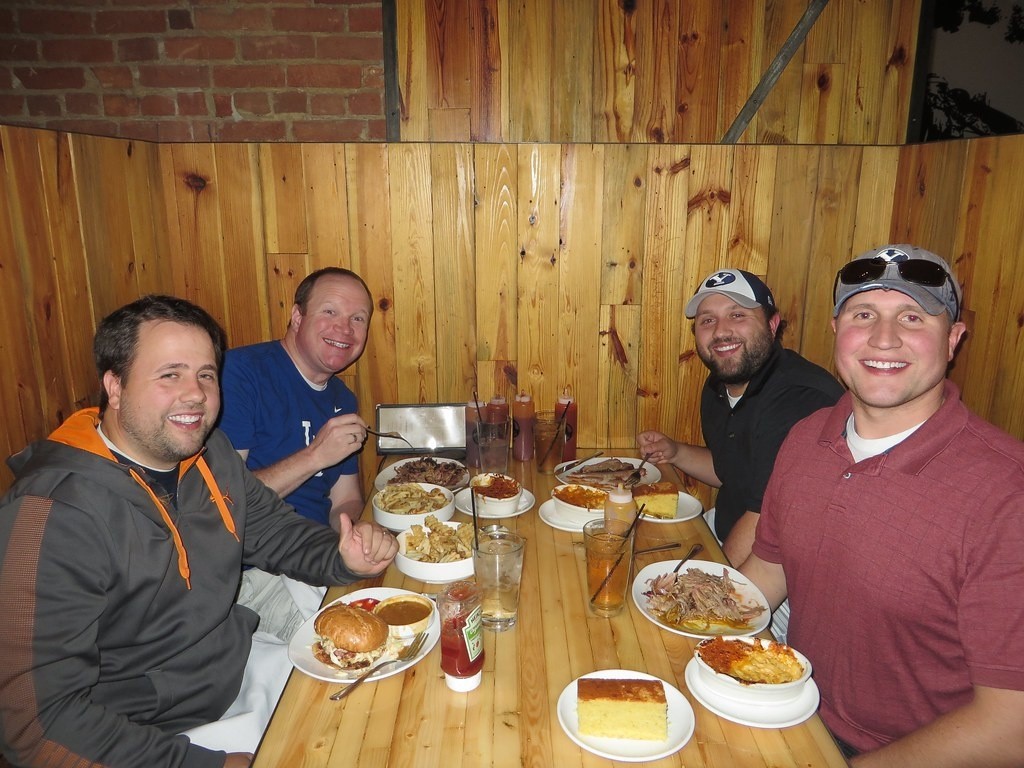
[351,433,357,443]
[383,531,391,535]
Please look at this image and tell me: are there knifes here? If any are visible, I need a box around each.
[554,452,604,475]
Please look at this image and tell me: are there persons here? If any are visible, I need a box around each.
[636,269,847,569]
[218,266,373,535]
[733,245,1024,768]
[0,293,401,768]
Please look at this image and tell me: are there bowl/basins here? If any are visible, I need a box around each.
[394,522,485,585]
[551,484,608,525]
[694,636,813,700]
[371,595,434,639]
[469,473,523,515]
[372,483,455,533]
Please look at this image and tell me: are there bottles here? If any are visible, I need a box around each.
[556,387,577,461]
[466,388,534,468]
[436,580,485,693]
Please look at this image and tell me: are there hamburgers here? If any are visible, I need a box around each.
[312,605,389,670]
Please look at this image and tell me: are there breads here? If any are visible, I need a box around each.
[578,678,668,742]
[631,482,680,517]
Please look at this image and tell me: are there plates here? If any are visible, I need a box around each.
[684,657,820,729]
[539,499,604,532]
[557,669,695,762]
[632,560,771,638]
[638,491,702,523]
[455,487,535,519]
[374,457,470,493]
[554,456,662,492]
[288,587,441,683]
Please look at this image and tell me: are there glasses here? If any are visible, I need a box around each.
[833,257,960,323]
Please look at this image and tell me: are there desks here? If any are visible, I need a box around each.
[251,450,850,768]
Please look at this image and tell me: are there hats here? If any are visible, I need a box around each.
[684,267,775,317]
[831,243,963,323]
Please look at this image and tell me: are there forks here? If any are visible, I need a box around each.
[329,633,430,700]
[365,427,414,449]
[625,453,653,486]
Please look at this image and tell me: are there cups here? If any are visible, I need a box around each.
[471,531,524,633]
[583,518,635,619]
[476,412,509,475]
[533,410,566,475]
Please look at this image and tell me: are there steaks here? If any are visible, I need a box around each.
[580,459,634,472]
